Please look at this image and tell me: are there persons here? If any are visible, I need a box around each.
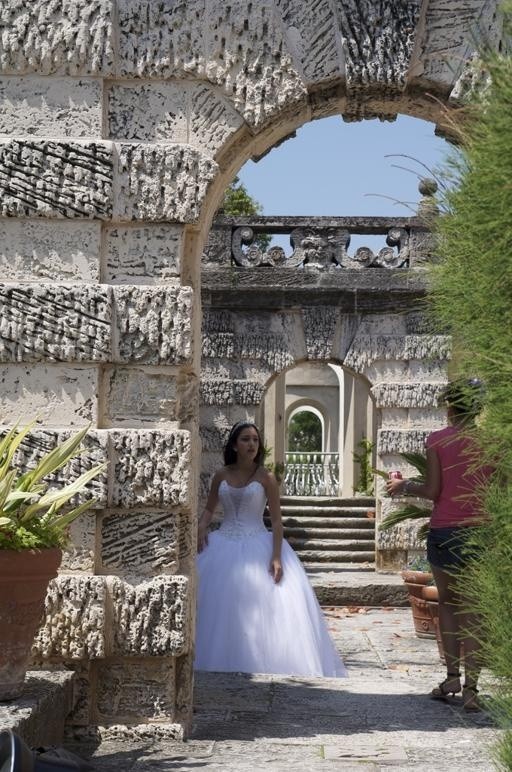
[195,419,350,679]
[385,377,503,712]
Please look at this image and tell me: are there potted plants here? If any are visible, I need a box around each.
[1,407,111,702]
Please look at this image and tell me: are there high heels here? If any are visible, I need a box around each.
[432,672,480,710]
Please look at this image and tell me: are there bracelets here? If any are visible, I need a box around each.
[402,479,415,496]
